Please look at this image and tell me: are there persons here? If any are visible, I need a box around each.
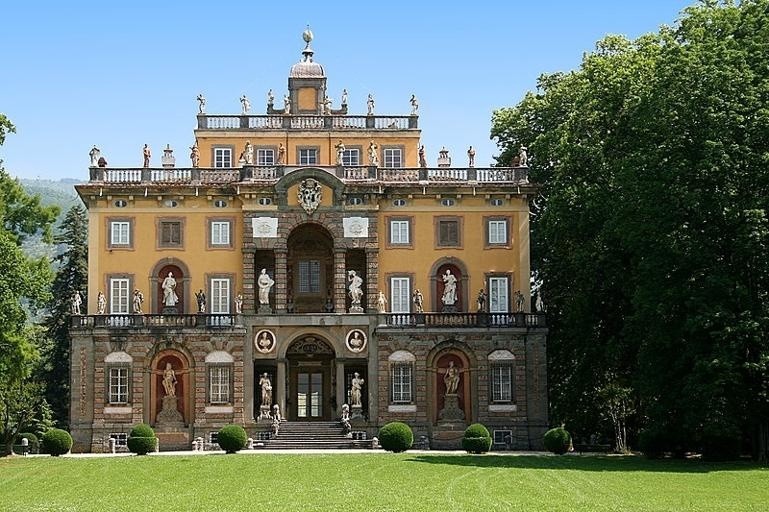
[324,95,333,114]
[440,269,459,307]
[413,287,425,313]
[160,362,179,398]
[476,287,488,313]
[341,87,349,104]
[517,141,528,167]
[408,93,418,116]
[267,87,274,104]
[258,373,273,407]
[529,277,544,313]
[195,288,206,314]
[162,271,179,304]
[466,145,476,167]
[334,139,346,166]
[243,139,254,165]
[89,142,101,167]
[367,92,375,114]
[70,289,83,315]
[96,290,109,314]
[349,371,365,406]
[239,94,251,113]
[347,269,364,306]
[143,142,151,167]
[234,294,243,315]
[514,290,525,312]
[257,267,275,305]
[375,289,388,314]
[442,360,460,395]
[197,93,206,114]
[131,288,144,315]
[418,144,427,167]
[366,139,380,167]
[283,93,291,113]
[276,141,286,165]
[189,141,200,167]
